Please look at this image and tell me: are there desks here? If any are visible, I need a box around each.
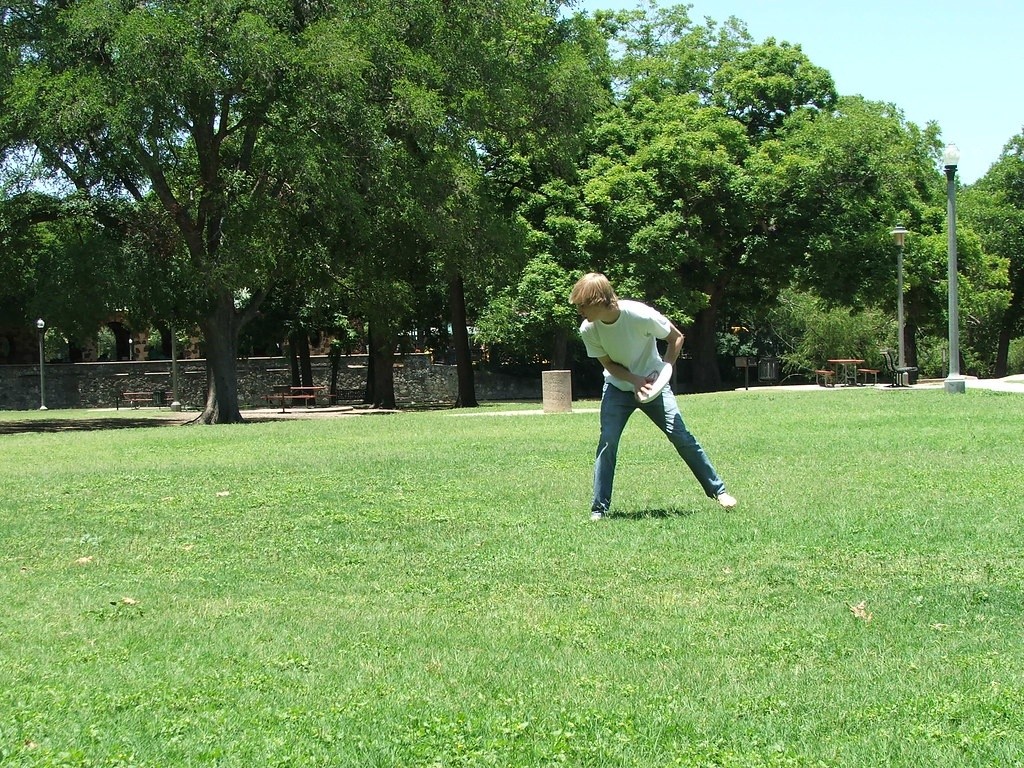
[828,359,865,387]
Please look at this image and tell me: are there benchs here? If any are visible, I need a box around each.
[261,384,336,408]
[879,349,919,387]
[816,371,833,387]
[858,369,879,387]
[122,391,173,408]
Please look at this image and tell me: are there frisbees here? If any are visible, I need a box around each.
[634,362,673,404]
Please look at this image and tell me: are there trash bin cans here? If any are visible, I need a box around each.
[757,357,779,386]
[153,390,167,407]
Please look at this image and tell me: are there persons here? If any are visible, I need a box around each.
[568,272,737,526]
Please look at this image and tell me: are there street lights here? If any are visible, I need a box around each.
[129,338,133,361]
[889,222,911,388]
[36,318,49,410]
[939,140,966,394]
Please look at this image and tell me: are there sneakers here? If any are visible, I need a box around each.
[718,493,736,507]
[590,511,604,521]
